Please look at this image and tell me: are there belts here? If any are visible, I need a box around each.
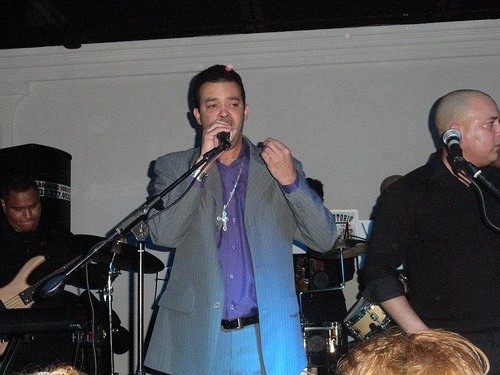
[221,313,259,329]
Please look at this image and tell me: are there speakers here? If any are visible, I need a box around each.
[0,144,72,234]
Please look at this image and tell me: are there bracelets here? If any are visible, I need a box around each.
[189,162,208,181]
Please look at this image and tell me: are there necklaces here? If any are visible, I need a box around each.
[216,155,246,232]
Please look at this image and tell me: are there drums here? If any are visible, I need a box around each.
[302,320,347,366]
[344,296,393,342]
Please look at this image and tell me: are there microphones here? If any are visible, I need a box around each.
[442,129,462,172]
[217,131,230,147]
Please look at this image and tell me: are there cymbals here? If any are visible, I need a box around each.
[307,240,366,259]
[67,233,164,275]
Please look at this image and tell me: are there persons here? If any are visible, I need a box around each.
[293,177,355,323]
[148,65,337,375]
[360,175,404,271]
[362,90,500,375]
[329,329,491,375]
[0,169,81,308]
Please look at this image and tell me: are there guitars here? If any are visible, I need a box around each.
[1,252,85,309]
